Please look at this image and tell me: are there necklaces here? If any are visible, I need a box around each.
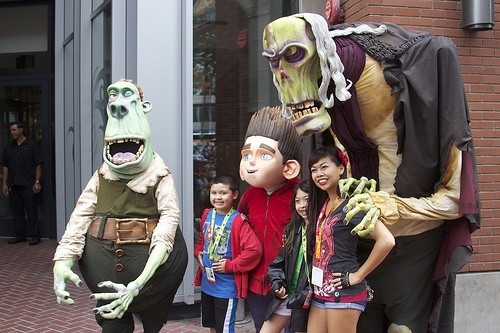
[209,206,234,260]
[316,195,342,262]
[301,222,310,286]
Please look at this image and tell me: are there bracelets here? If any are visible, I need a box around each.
[34,180,41,183]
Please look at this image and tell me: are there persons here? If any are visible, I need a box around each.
[191,174,263,333]
[307,146,396,333]
[258,180,311,333]
[2,121,43,245]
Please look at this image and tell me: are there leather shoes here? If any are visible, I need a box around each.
[8,237,27,244]
[29,237,40,244]
